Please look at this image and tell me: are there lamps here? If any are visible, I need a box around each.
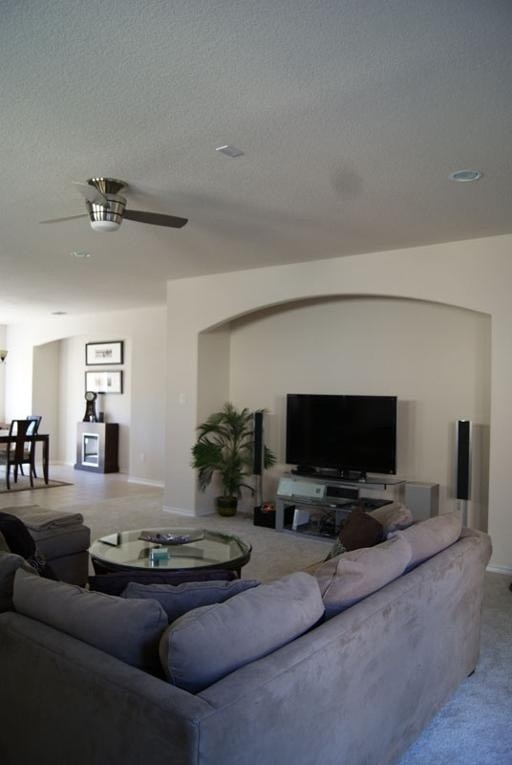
[1,349,9,361]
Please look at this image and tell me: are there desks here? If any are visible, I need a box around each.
[0,424,51,485]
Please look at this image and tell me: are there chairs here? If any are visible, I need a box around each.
[26,414,43,434]
[7,420,40,488]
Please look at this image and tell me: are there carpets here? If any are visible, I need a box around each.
[0,468,73,493]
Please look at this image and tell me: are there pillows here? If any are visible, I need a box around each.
[14,560,170,656]
[324,537,412,609]
[119,573,260,611]
[163,570,325,678]
[399,509,471,570]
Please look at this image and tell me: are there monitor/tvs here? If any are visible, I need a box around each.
[283,393,397,482]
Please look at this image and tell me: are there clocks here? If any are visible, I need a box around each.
[82,391,98,422]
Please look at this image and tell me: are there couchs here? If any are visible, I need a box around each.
[2,499,494,765]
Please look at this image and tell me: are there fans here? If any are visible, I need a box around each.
[37,175,189,233]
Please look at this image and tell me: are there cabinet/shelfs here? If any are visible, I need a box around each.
[74,421,119,474]
[278,473,394,541]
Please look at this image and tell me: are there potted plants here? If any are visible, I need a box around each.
[190,401,278,518]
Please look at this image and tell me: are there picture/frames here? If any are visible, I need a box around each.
[84,371,124,394]
[84,341,125,364]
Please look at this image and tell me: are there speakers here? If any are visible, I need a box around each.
[253,410,263,477]
[455,417,472,502]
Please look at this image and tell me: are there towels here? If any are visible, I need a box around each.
[0,503,85,531]
